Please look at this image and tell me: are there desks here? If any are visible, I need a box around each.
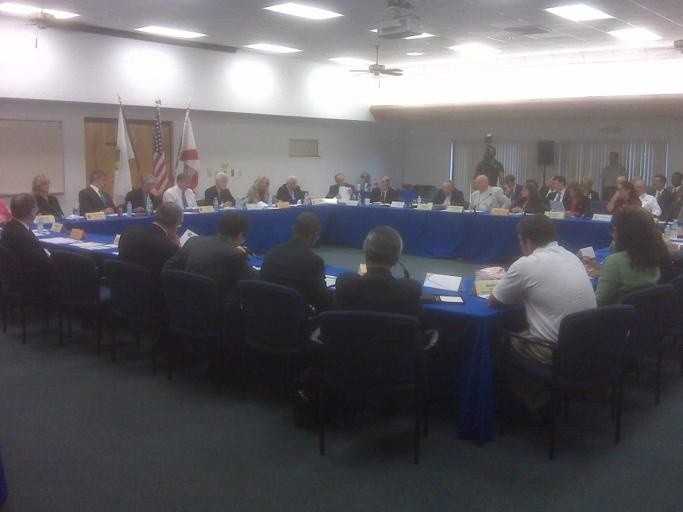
[11,196,682,448]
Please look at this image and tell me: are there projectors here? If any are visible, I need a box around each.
[377,14,424,40]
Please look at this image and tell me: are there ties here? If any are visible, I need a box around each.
[291,189,294,200]
[219,191,223,204]
[182,191,188,209]
[99,190,109,206]
[673,186,677,194]
[381,191,385,203]
[556,191,560,202]
[657,192,661,203]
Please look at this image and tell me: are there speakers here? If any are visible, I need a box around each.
[538,140,554,163]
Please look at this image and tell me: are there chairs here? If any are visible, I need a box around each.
[303,310,440,466]
[154,267,311,412]
[13,257,161,366]
[498,276,680,461]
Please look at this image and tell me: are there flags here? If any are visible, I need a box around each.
[111,109,134,207]
[174,110,201,201]
[148,107,171,198]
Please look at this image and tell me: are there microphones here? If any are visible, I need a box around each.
[192,189,214,210]
[242,193,268,203]
[474,193,493,211]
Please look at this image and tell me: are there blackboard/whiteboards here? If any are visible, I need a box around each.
[0,118,65,196]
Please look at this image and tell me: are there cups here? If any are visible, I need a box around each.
[117,208,123,216]
[364,198,370,205]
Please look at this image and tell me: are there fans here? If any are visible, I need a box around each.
[5,0,83,36]
[346,44,406,79]
[639,36,683,60]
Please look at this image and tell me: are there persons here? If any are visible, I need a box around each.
[204,173,235,207]
[31,175,62,216]
[486,213,598,426]
[162,174,196,209]
[159,209,257,332]
[600,152,626,200]
[76,170,118,215]
[580,204,660,307]
[276,178,302,204]
[475,147,503,187]
[318,225,434,423]
[124,174,163,213]
[244,175,271,206]
[117,202,181,315]
[259,211,330,431]
[0,193,56,305]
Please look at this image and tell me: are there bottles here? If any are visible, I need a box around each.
[268,193,273,207]
[146,192,152,216]
[664,218,678,240]
[416,195,421,204]
[126,200,133,217]
[74,201,80,220]
[36,212,43,233]
[213,197,218,212]
[304,192,308,208]
